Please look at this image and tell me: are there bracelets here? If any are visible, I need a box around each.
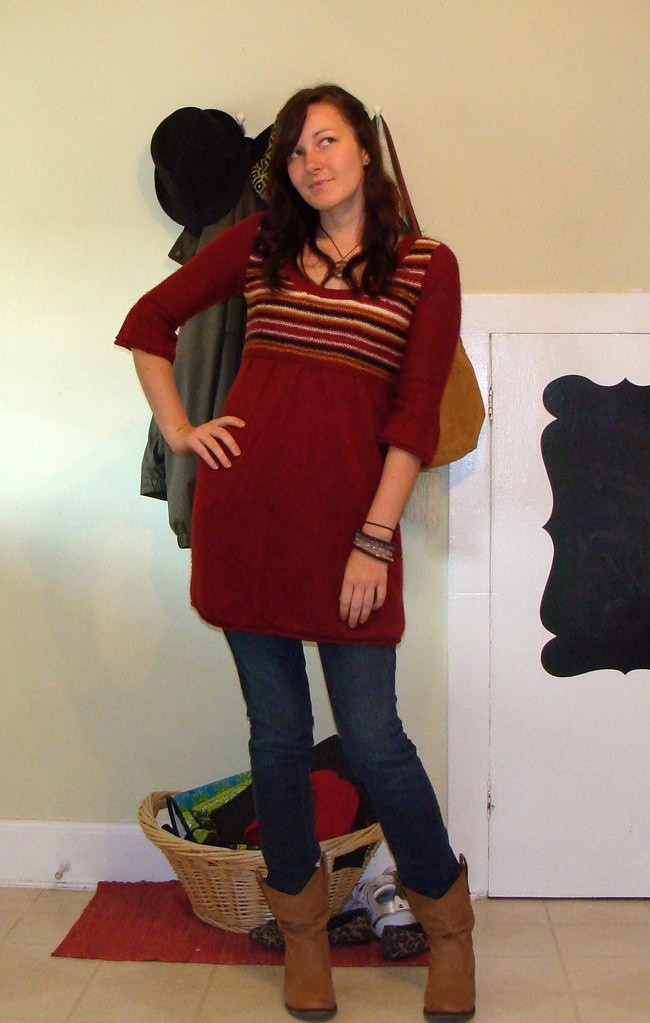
[364,520,394,534]
[352,528,396,564]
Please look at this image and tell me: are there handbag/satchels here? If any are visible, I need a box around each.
[371,115,484,470]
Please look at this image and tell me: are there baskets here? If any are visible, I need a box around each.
[137,790,385,935]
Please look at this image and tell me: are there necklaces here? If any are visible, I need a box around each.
[319,223,363,280]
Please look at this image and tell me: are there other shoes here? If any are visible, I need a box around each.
[380,923,431,961]
[249,907,374,950]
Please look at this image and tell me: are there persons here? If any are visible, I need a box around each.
[115,82,480,1023]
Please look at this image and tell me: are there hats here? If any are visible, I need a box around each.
[250,116,275,207]
[150,105,250,226]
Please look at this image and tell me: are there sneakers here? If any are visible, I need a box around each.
[343,865,417,937]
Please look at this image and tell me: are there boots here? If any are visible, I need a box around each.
[392,853,475,1021]
[253,851,339,1020]
